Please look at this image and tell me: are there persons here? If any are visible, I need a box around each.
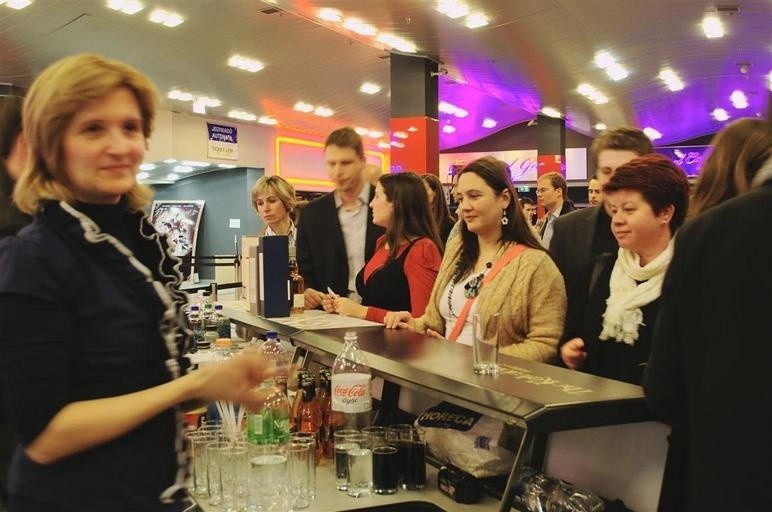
[550,118,772,512]
[252,127,575,362]
[0,93,34,238]
[0,50,297,512]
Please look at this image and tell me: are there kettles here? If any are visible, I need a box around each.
[189,288,228,348]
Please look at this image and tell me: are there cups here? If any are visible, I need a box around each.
[470,310,503,376]
[182,423,430,512]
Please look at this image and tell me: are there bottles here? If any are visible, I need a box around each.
[247,377,291,465]
[331,331,372,432]
[257,331,292,423]
[213,337,234,367]
[287,245,305,314]
[291,366,342,466]
